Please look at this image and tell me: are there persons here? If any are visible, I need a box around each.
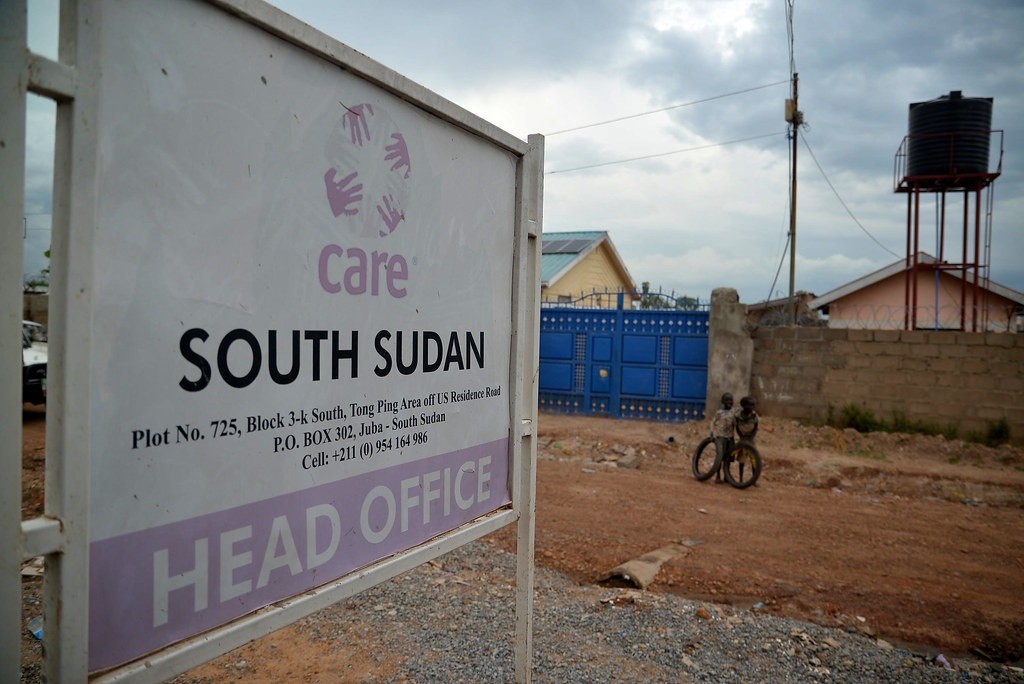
[710,392,763,488]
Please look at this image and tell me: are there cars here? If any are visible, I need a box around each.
[21,321,47,405]
